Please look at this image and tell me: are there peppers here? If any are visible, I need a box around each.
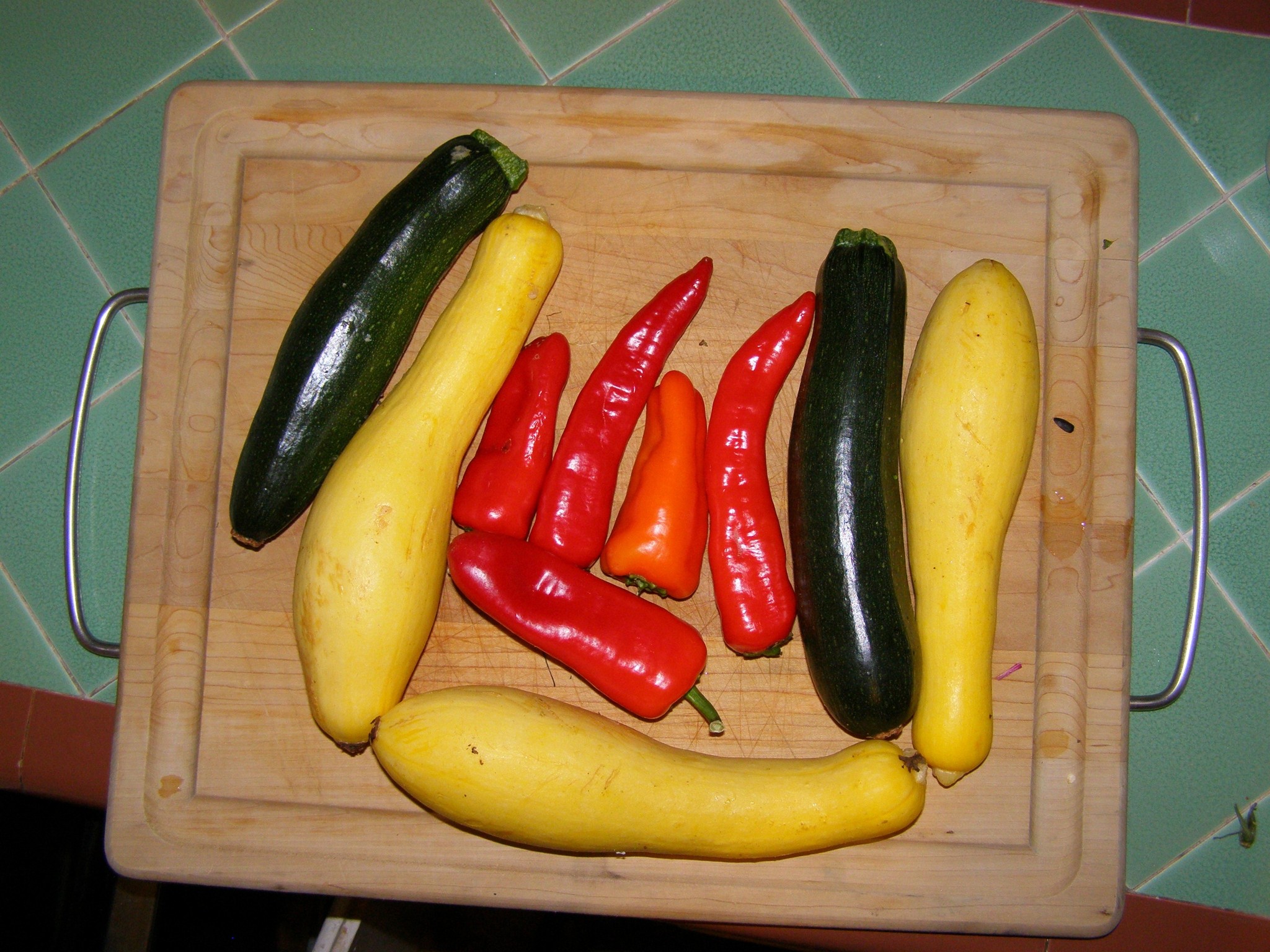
[448,258,815,735]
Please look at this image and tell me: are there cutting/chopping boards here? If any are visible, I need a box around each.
[64,82,1212,940]
